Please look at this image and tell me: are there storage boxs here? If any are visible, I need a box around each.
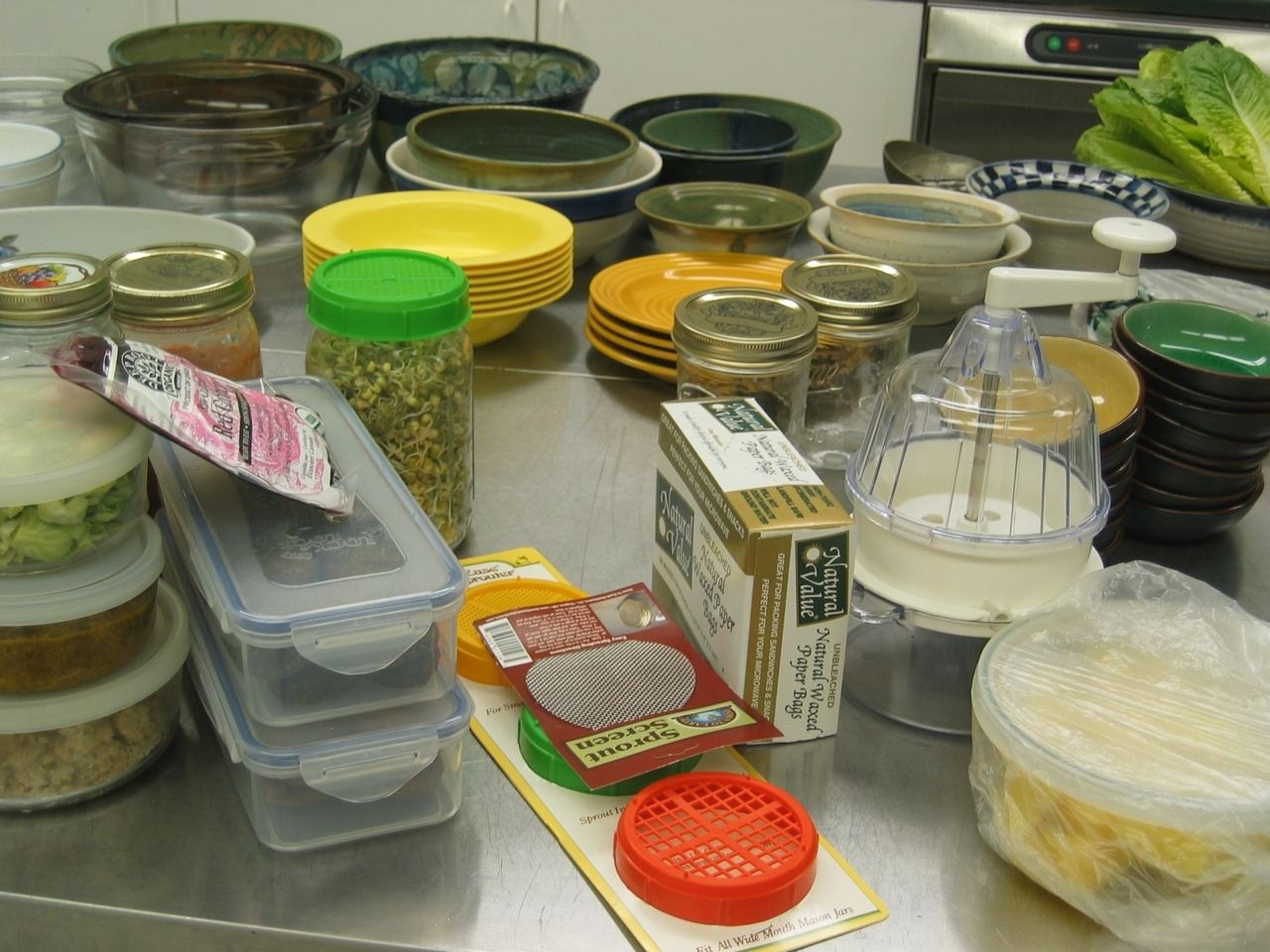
[145,370,465,725]
[653,396,853,749]
[188,613,475,855]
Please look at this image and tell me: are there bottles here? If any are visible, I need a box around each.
[0,251,130,392]
[104,242,262,382]
[304,251,472,553]
[673,254,919,471]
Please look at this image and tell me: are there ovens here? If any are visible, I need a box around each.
[910,0,1270,166]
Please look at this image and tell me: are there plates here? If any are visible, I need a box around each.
[586,252,797,386]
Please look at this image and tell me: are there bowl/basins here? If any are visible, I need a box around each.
[610,94,841,198]
[972,600,1270,910]
[62,60,378,267]
[883,138,983,187]
[0,55,105,209]
[0,206,255,255]
[387,131,662,265]
[805,183,1033,325]
[406,104,638,191]
[1139,178,1270,270]
[0,368,192,813]
[341,36,600,172]
[303,190,573,346]
[967,159,1168,273]
[633,184,812,254]
[937,335,1145,568]
[106,20,343,64]
[1110,299,1270,541]
[148,376,475,851]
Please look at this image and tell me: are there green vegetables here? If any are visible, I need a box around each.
[1074,42,1270,208]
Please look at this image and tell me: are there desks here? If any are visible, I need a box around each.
[0,160,1270,952]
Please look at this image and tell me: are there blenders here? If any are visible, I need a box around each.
[845,214,1177,735]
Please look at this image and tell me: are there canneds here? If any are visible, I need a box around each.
[107,243,265,381]
[671,287,817,462]
[0,252,124,358]
[304,249,478,552]
[780,253,920,469]
